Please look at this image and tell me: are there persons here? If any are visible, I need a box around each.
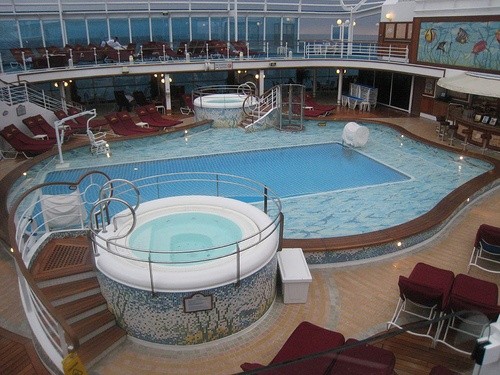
[107,35,127,64]
[99,40,109,64]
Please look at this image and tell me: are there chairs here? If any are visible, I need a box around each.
[467,224,500,274]
[239,321,346,375]
[0,38,338,160]
[328,338,396,375]
[431,273,500,356]
[429,365,461,375]
[386,262,455,348]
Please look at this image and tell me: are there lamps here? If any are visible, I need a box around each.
[386,13,392,21]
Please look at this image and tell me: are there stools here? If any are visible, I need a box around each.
[448,125,458,147]
[440,121,450,142]
[460,129,473,150]
[479,133,492,155]
[436,115,446,136]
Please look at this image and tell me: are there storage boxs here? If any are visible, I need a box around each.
[277,248,312,303]
